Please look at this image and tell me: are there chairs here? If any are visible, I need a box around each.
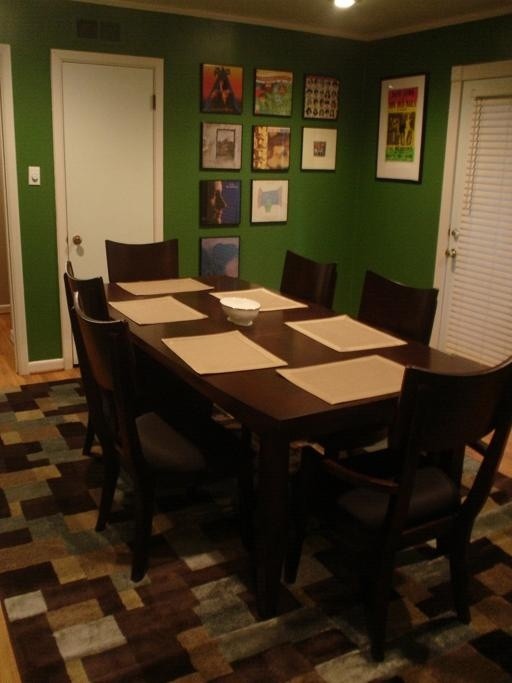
[283,355,511,647]
[278,248,339,309]
[71,289,264,582]
[64,260,111,456]
[356,270,439,347]
[104,237,180,285]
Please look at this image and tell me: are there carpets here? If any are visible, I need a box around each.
[0,375,512,678]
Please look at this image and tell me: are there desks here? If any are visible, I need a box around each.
[101,274,497,577]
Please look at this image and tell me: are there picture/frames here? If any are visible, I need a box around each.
[374,71,429,184]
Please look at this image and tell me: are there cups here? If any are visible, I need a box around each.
[220,296,262,328]
